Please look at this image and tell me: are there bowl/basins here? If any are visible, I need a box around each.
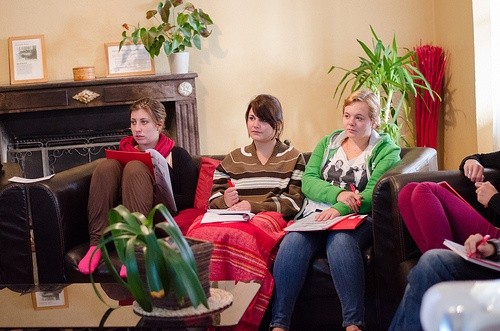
[72,66,96,81]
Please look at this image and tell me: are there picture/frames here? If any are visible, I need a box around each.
[8,35,47,84]
[32,287,69,309]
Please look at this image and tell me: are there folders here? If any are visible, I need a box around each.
[328,214,368,230]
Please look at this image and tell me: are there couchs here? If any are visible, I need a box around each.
[372,169,496,331]
[0,163,35,283]
[29,148,437,331]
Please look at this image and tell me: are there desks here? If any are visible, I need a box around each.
[0,280,262,329]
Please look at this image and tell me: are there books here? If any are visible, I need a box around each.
[443,239,500,271]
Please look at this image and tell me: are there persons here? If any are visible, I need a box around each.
[268,89,401,331]
[185,95,306,331]
[78,96,197,277]
[398,151,500,255]
[389,234,500,331]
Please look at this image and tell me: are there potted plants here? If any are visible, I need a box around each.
[119,0,213,74]
[91,204,213,313]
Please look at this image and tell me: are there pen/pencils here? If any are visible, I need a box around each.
[350,184,362,206]
[468,235,491,258]
[227,180,238,204]
